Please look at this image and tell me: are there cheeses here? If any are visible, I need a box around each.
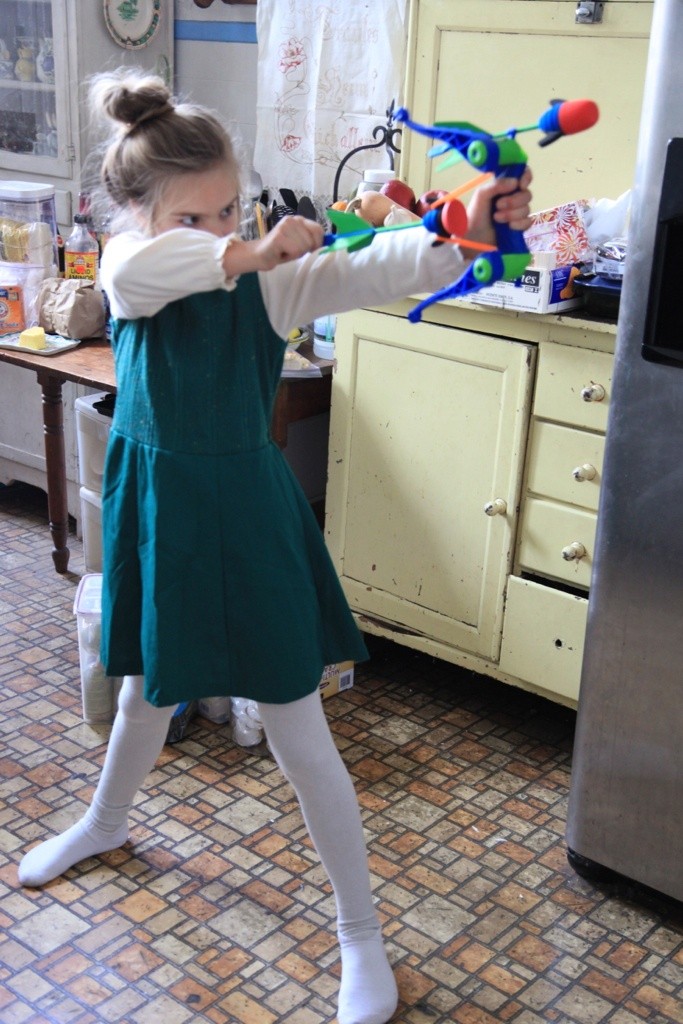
[19,326,46,350]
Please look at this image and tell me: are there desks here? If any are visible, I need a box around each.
[0,323,334,583]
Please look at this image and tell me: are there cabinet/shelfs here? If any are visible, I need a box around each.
[324,289,623,715]
[0,0,179,240]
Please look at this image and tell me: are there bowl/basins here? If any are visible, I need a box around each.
[573,272,623,319]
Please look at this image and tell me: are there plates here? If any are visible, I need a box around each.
[287,327,310,350]
[104,0,162,50]
[0,331,81,355]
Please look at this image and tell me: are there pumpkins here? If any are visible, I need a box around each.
[345,191,423,230]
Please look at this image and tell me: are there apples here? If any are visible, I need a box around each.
[379,180,451,219]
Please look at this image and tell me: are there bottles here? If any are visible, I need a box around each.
[314,314,335,360]
[105,296,112,341]
[79,192,97,240]
[41,203,65,272]
[65,215,100,290]
[357,171,396,194]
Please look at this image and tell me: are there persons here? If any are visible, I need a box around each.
[17,71,537,1024]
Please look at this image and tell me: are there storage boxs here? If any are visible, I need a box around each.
[76,486,107,573]
[316,661,357,702]
[70,562,129,727]
[0,180,64,285]
[449,200,597,318]
[0,285,34,339]
[70,385,121,496]
